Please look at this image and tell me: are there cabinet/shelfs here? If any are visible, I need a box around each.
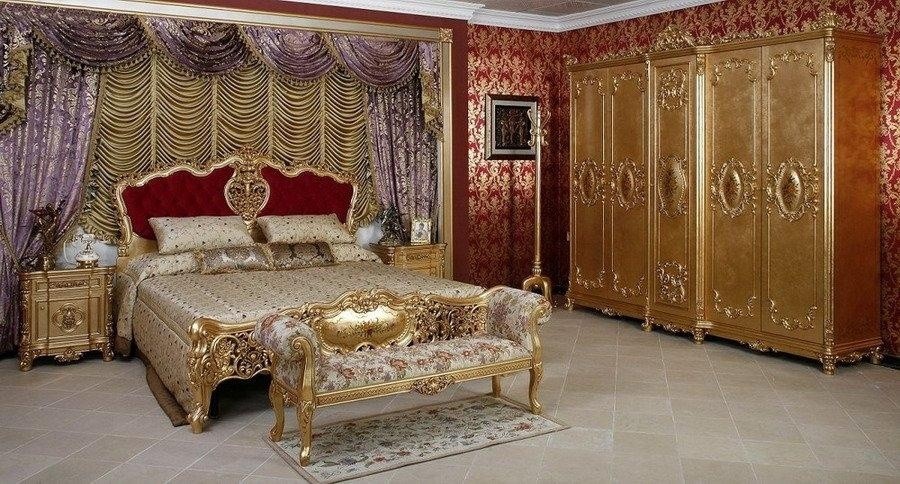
[562,10,885,375]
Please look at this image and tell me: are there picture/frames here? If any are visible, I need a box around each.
[409,216,432,246]
[484,93,540,162]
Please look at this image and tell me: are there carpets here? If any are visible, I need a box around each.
[261,393,572,484]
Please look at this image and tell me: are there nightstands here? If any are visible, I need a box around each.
[368,242,449,278]
[17,266,117,371]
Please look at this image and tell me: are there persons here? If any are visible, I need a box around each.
[414,223,428,240]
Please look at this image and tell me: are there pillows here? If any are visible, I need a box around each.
[147,212,357,276]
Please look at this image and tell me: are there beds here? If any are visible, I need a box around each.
[109,140,490,436]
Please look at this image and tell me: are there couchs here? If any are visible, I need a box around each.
[255,284,553,467]
